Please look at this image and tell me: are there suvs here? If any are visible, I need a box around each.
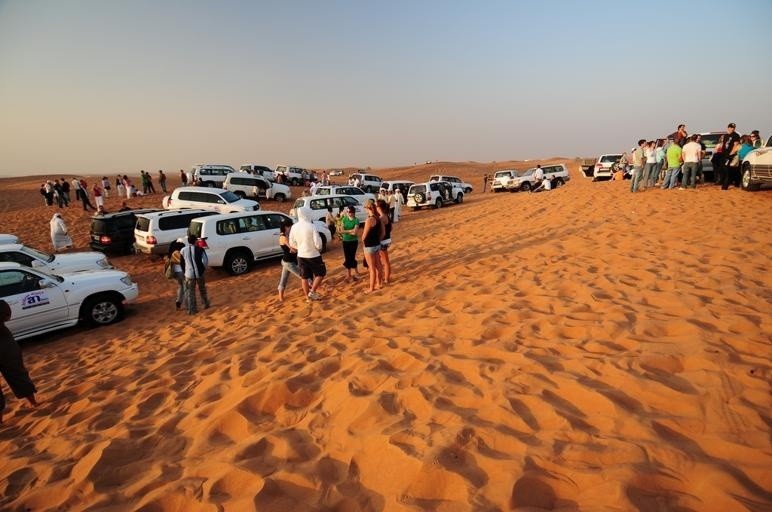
[740,135,772,190]
[506,163,569,192]
[0,263,141,344]
[491,169,520,193]
[652,131,741,183]
[578,154,626,182]
[0,232,116,275]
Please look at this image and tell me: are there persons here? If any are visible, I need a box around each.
[620,123,761,195]
[180,170,189,185]
[277,170,405,301]
[0,299,38,423]
[483,174,488,192]
[50,212,74,252]
[168,237,189,310]
[529,164,558,193]
[179,235,211,316]
[40,167,168,215]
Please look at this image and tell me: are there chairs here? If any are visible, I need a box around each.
[218,218,260,234]
[336,200,341,207]
